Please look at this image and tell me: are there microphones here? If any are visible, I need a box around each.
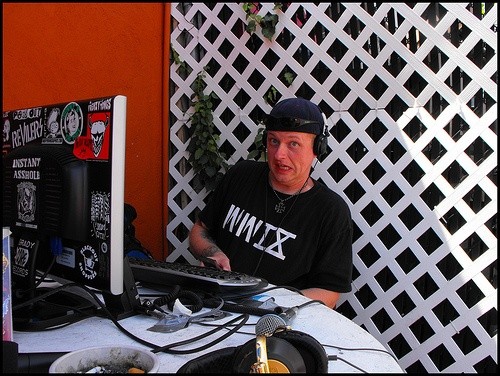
[1,341,71,376]
[255,306,301,337]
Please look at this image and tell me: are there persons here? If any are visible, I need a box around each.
[124,204,162,292]
[189,98,352,309]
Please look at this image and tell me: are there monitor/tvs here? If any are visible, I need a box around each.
[3,94,127,332]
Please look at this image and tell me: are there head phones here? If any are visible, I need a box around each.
[262,104,330,155]
[175,326,328,374]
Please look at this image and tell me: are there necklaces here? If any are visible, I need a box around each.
[270,180,309,213]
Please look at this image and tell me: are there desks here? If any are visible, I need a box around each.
[0,267,403,376]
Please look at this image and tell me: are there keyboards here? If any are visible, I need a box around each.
[127,257,268,295]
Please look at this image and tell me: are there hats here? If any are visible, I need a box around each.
[266,98,323,135]
